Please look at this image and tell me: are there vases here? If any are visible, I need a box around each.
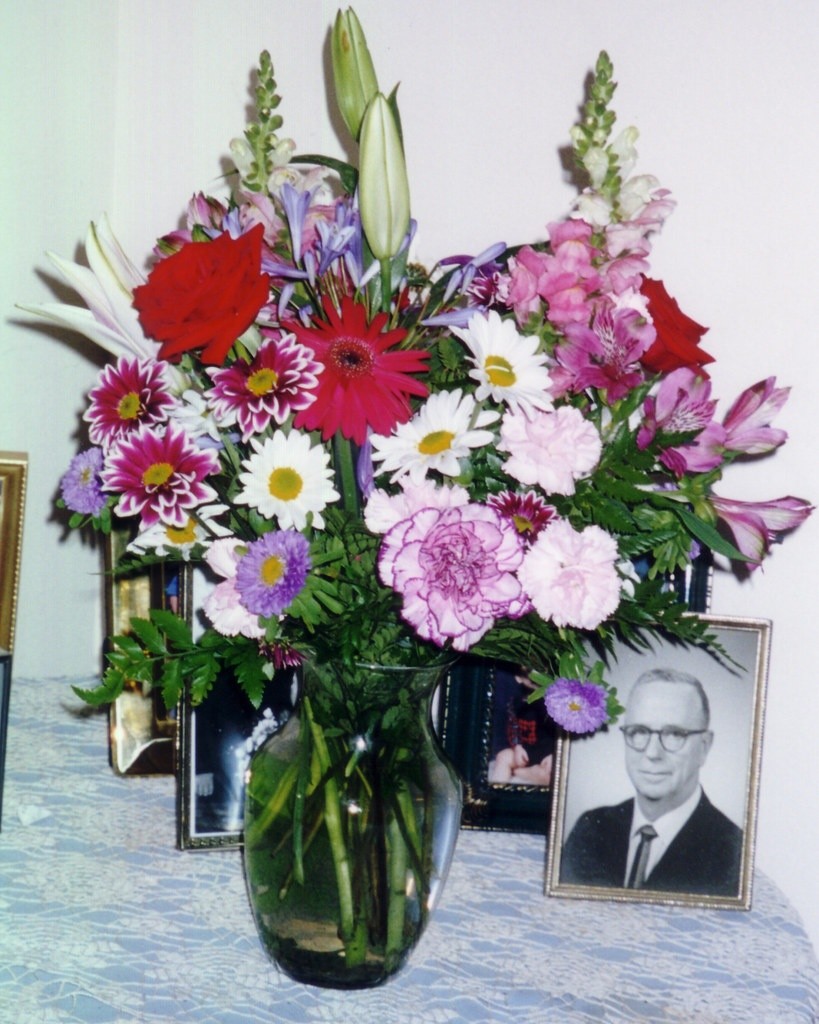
[244,647,465,990]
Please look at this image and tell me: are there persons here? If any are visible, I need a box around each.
[487,667,557,787]
[560,667,746,897]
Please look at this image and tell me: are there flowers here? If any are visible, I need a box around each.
[13,6,815,969]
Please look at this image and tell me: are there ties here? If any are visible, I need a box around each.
[627,826,658,889]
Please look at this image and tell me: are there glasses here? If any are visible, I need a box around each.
[620,726,707,752]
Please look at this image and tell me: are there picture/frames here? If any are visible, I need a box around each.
[0,450,30,651]
[440,490,713,835]
[174,559,301,852]
[545,613,774,911]
[104,501,182,778]
